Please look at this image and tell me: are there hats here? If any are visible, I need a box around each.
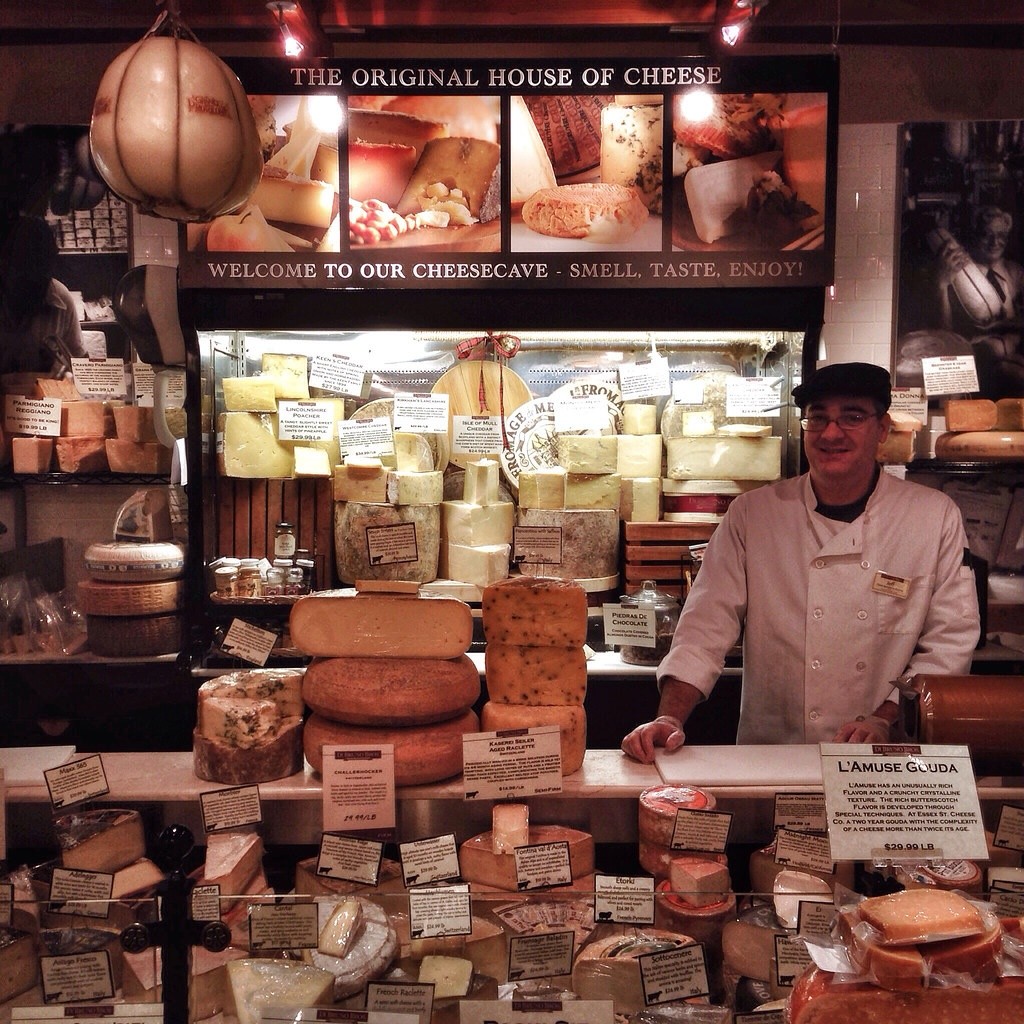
[791,363,891,407]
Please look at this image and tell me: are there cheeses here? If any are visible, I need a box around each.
[201,87,828,230]
[0,353,1024,1024]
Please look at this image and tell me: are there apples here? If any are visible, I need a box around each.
[207,211,261,251]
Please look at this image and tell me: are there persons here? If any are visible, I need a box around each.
[621,362,981,766]
[931,204,1024,363]
[0,216,91,375]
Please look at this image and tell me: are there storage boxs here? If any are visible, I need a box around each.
[460,826,594,892]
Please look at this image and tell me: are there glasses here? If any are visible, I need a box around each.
[800,411,878,431]
